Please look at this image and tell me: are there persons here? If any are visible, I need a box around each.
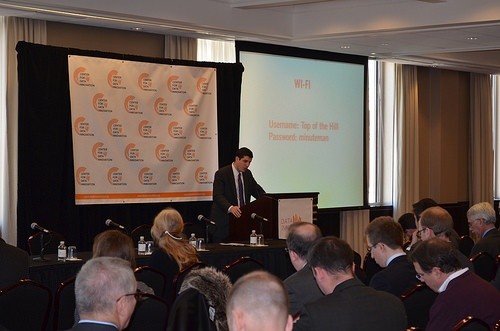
[282,221,325,317]
[294,236,408,331]
[209,147,267,242]
[0,237,29,293]
[226,270,293,331]
[366,216,417,297]
[466,202,500,260]
[149,208,209,281]
[72,230,154,325]
[67,256,140,331]
[398,198,459,248]
[408,237,500,331]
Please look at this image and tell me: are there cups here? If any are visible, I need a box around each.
[147,241,155,254]
[257,235,264,246]
[197,238,205,250]
[68,246,77,260]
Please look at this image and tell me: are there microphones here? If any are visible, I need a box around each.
[198,215,215,225]
[251,213,268,221]
[31,223,49,232]
[105,219,124,229]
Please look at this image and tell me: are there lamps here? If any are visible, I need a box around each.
[128,25,148,34]
[431,64,439,68]
[337,44,352,51]
[463,36,480,41]
[377,42,392,49]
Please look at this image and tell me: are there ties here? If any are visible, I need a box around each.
[238,172,244,207]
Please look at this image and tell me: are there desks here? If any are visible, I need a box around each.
[32,240,295,285]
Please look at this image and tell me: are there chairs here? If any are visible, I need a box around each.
[54,274,77,331]
[172,260,215,295]
[0,279,53,331]
[290,234,500,331]
[222,255,269,286]
[129,262,174,331]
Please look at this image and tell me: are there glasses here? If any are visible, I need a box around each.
[367,241,384,251]
[416,228,433,239]
[285,248,297,253]
[416,268,440,282]
[468,219,485,228]
[116,291,142,302]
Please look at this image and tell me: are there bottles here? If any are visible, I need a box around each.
[138,236,146,255]
[189,233,197,248]
[250,230,258,245]
[57,241,66,262]
